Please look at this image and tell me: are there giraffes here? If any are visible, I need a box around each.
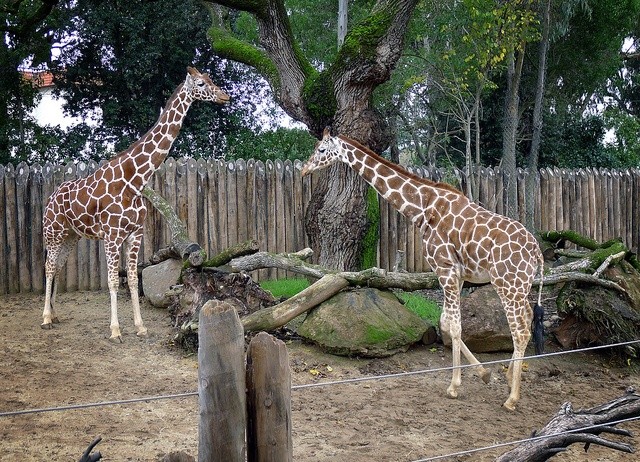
[40,66,232,344]
[301,125,545,412]
